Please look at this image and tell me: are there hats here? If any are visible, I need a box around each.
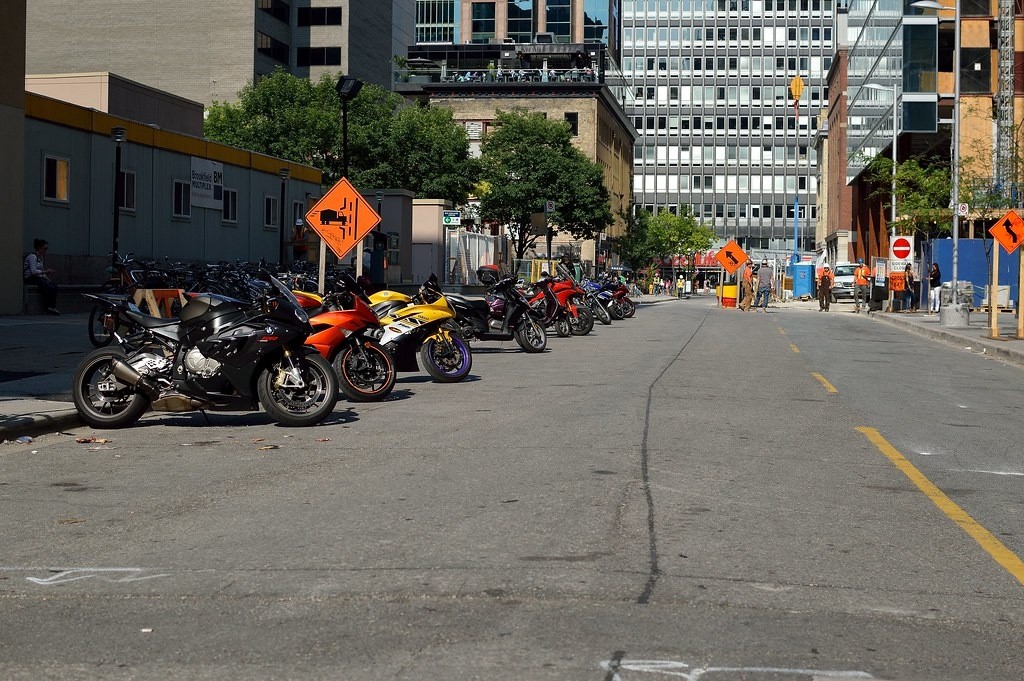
[295,219,303,225]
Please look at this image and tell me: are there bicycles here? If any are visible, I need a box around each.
[89,249,346,348]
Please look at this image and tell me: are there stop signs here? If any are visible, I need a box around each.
[889,236,914,291]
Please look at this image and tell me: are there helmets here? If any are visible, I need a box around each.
[763,260,767,264]
[654,273,658,276]
[746,259,751,264]
[679,275,683,278]
[823,263,829,267]
[858,258,863,263]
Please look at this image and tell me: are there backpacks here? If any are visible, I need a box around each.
[22,248,41,270]
[363,248,383,281]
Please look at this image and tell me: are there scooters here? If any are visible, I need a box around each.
[419,265,547,353]
[476,265,570,337]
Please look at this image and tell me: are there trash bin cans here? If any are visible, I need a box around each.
[648,285,654,295]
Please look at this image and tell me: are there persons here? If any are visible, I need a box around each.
[904,263,917,314]
[676,275,685,301]
[538,271,549,283]
[695,279,699,296]
[738,260,754,312]
[752,259,774,313]
[926,262,941,314]
[653,273,673,298]
[818,262,834,312]
[602,269,648,300]
[558,254,577,281]
[854,258,871,310]
[22,237,61,315]
[784,275,793,303]
[714,282,720,306]
[703,278,712,296]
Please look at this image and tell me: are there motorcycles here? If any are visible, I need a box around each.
[71,275,339,429]
[292,272,472,383]
[530,276,635,336]
[182,274,396,401]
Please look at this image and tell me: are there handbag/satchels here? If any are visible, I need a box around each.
[37,272,57,288]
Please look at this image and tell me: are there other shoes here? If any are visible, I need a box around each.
[825,308,829,311]
[927,310,933,313]
[819,307,823,311]
[935,310,940,313]
[739,304,744,311]
[762,309,766,313]
[48,306,63,313]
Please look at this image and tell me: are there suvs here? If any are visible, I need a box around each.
[831,263,871,303]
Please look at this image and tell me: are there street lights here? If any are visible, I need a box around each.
[862,84,897,313]
[279,167,292,264]
[110,127,128,267]
[910,0,970,326]
